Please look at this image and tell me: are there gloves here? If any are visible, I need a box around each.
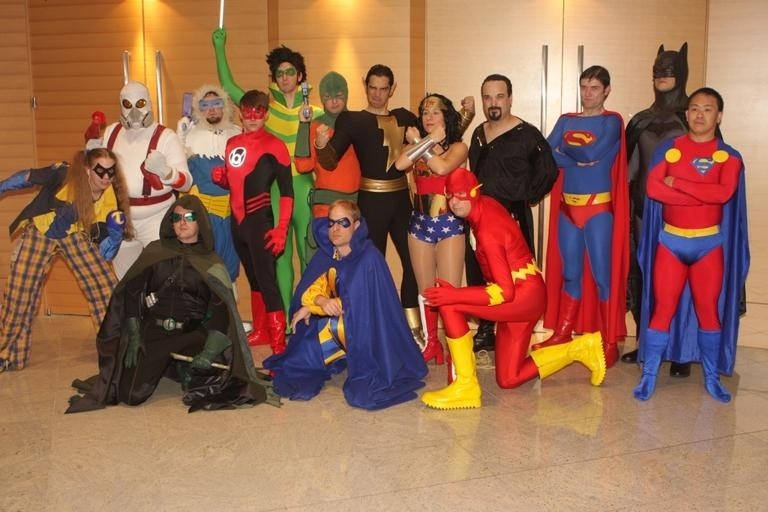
[124,316,147,369]
[192,329,232,369]
[144,150,171,179]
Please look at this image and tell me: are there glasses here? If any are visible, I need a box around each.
[172,211,197,223]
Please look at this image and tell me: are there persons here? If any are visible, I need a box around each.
[0,147,129,369]
[176,83,294,354]
[624,44,692,379]
[633,88,744,403]
[213,30,325,334]
[262,201,430,412]
[467,73,560,353]
[85,81,192,281]
[421,168,606,410]
[64,191,274,411]
[545,64,623,369]
[293,64,476,351]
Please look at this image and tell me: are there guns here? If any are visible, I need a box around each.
[300,80,310,122]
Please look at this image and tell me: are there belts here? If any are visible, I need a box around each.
[155,318,182,330]
